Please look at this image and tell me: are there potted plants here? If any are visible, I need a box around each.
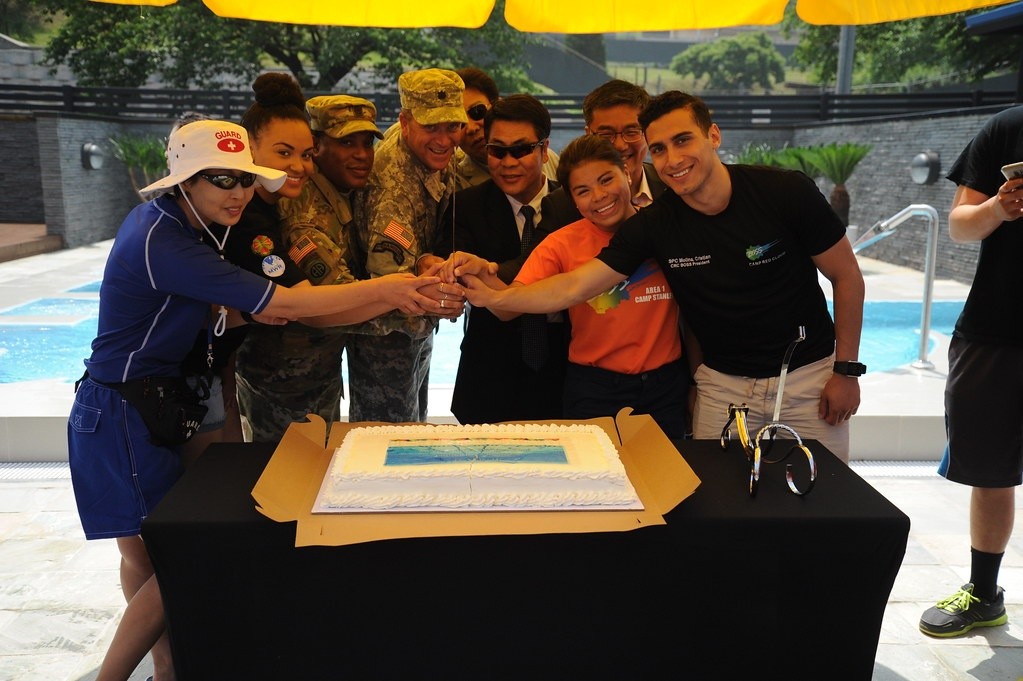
[801,142,875,247]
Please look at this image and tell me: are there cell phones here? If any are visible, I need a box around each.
[1001,162,1023,190]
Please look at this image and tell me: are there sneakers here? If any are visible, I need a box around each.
[919,583,1008,637]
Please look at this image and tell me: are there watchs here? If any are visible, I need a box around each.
[832,360,866,378]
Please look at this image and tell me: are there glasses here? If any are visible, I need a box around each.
[461,102,489,130]
[588,127,644,145]
[201,172,257,191]
[485,140,542,160]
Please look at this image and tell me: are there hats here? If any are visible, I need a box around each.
[397,68,470,125]
[306,95,384,141]
[138,119,286,198]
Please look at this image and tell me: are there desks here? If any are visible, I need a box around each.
[143,438,913,680]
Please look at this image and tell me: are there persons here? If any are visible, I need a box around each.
[234,93,468,445]
[455,90,866,468]
[489,80,667,276]
[179,71,442,458]
[416,93,571,420]
[347,67,475,423]
[919,105,1023,638]
[67,119,439,681]
[438,136,702,438]
[448,70,559,188]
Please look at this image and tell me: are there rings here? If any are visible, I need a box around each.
[440,283,443,292]
[443,293,447,300]
[441,300,444,308]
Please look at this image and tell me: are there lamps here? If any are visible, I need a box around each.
[84,142,104,170]
[910,152,940,185]
